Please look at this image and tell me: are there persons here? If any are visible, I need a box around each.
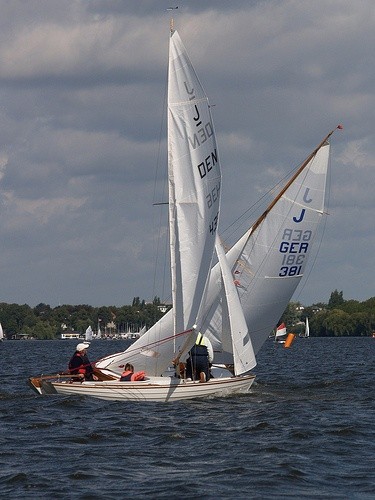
[69,343,99,382]
[119,363,145,381]
[186,344,210,383]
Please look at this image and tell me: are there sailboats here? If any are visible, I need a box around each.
[25,8,344,403]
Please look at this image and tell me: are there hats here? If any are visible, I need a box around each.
[76,342,90,352]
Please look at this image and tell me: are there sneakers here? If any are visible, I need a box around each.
[200,372,206,382]
[186,378,192,381]
